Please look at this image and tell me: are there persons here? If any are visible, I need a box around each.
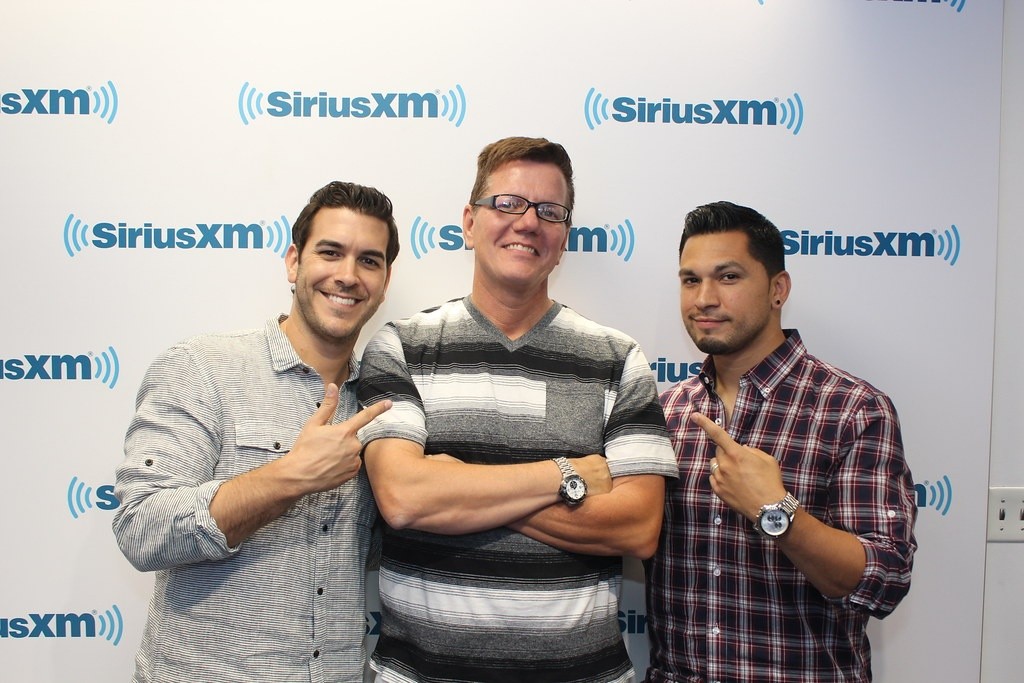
[754,489,798,540]
[554,455,587,506]
[630,201,922,683]
[111,181,391,681]
[357,136,681,681]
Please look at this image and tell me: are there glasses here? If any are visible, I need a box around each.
[473,193,572,223]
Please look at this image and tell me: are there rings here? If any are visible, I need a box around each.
[709,462,719,476]
